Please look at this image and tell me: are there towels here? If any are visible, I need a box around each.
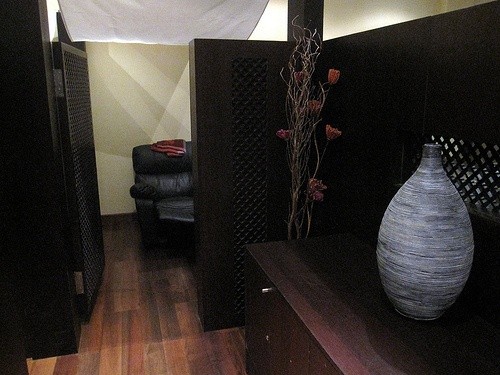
[149,138,188,158]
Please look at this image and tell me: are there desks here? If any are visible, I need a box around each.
[244,232,500,375]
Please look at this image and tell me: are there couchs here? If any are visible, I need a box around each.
[130,141,193,245]
